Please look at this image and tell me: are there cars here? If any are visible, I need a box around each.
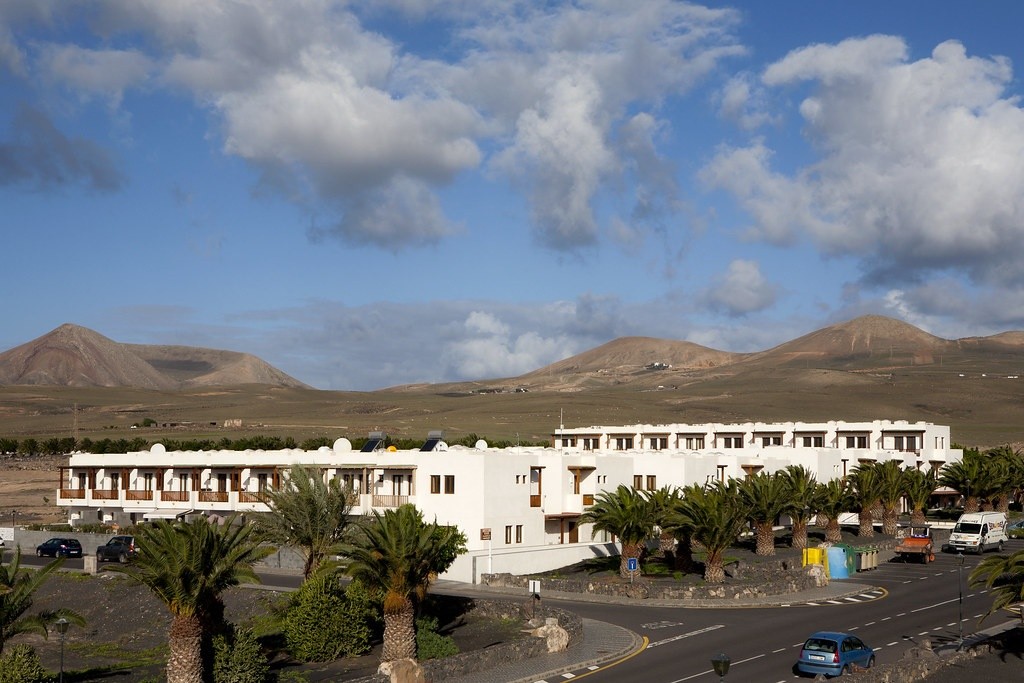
[36,538,82,558]
[96,536,150,564]
[1008,520,1024,539]
[797,631,875,677]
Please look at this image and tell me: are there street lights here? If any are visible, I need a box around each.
[12,510,16,527]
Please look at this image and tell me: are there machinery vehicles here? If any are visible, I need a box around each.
[894,524,935,564]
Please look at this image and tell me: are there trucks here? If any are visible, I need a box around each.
[949,512,1008,555]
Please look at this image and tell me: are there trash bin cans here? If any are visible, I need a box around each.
[802,544,856,580]
[853,546,879,572]
